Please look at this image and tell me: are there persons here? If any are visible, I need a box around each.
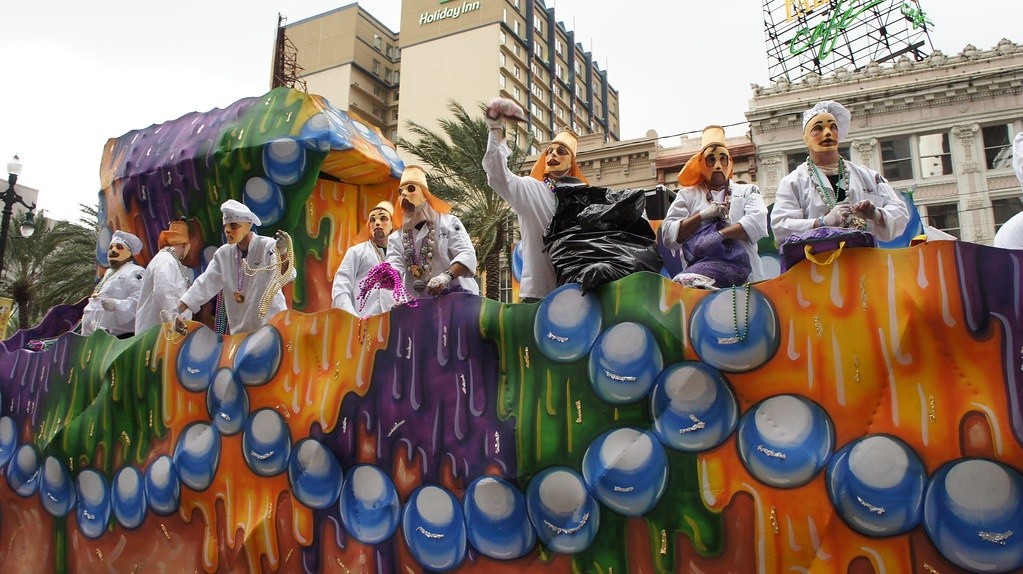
[659,126,769,287]
[169,199,297,337]
[330,200,400,317]
[770,100,911,242]
[81,230,147,339]
[481,110,590,304]
[134,217,204,336]
[993,131,1023,250]
[385,164,479,299]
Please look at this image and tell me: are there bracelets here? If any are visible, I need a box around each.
[444,270,455,280]
[818,216,825,226]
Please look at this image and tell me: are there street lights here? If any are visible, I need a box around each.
[0,153,36,272]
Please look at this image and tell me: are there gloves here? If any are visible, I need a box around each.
[699,203,728,220]
[275,230,289,255]
[426,272,451,296]
[485,97,526,131]
[850,199,881,224]
[819,202,850,227]
[101,299,115,311]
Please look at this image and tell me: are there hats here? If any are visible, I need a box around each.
[803,100,852,141]
[158,220,190,250]
[352,200,395,244]
[220,200,262,227]
[110,230,143,256]
[678,125,734,186]
[529,132,590,185]
[391,165,452,229]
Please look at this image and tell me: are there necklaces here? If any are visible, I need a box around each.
[94,265,121,294]
[235,245,248,291]
[169,248,192,290]
[356,261,419,312]
[213,289,231,336]
[805,153,850,212]
[732,282,749,341]
[542,173,560,206]
[159,309,185,344]
[358,318,368,343]
[403,223,435,292]
[243,233,295,321]
